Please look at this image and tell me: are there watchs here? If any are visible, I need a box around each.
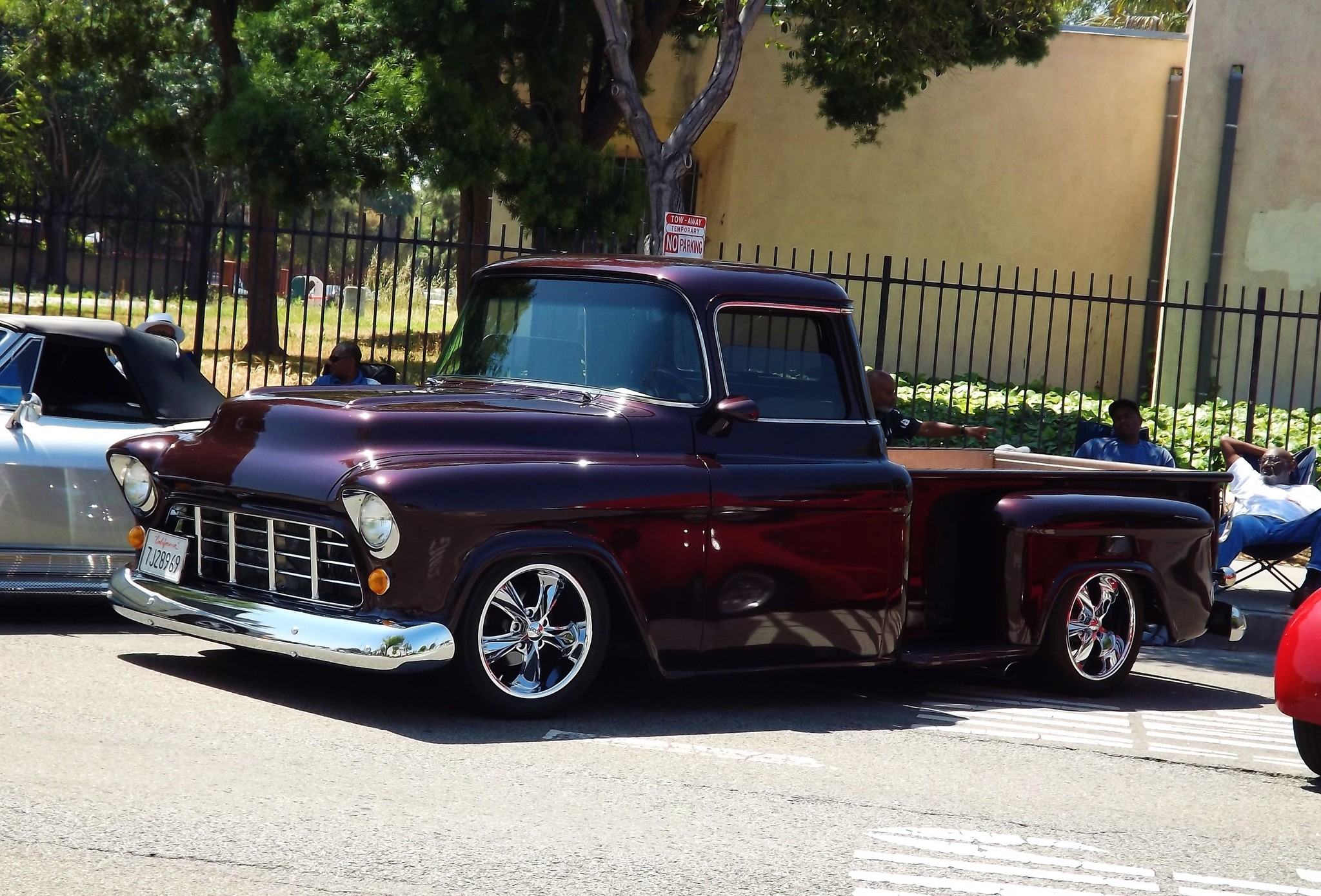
[961,424,968,435]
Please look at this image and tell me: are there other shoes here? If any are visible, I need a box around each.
[1294,586,1317,605]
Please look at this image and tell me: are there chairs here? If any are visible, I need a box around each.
[1215,447,1317,603]
[1071,419,1149,461]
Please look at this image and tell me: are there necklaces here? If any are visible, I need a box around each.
[1275,482,1291,489]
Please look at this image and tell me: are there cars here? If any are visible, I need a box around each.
[207,271,248,298]
[1,313,228,594]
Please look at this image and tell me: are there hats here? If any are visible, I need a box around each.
[1108,399,1141,419]
[136,313,185,344]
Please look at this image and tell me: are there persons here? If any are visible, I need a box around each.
[1073,399,1175,468]
[135,313,185,344]
[866,367,996,447]
[311,341,381,386]
[1215,436,1321,609]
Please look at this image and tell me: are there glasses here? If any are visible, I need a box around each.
[150,333,176,341]
[329,355,354,363]
[1258,456,1290,465]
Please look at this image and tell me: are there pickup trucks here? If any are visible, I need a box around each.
[104,252,1249,725]
